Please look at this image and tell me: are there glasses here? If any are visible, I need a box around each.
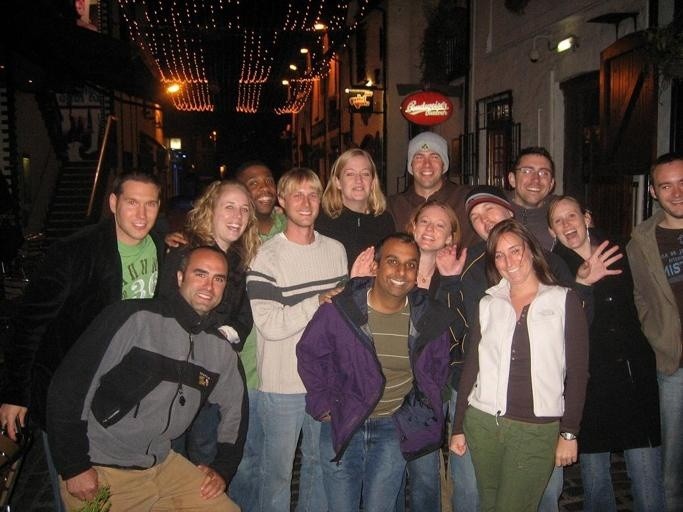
[514,167,551,178]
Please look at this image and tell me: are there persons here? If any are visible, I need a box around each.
[1,132,662,511]
[625,152,683,511]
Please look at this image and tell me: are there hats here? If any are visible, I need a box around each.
[464,185,515,234]
[408,132,448,175]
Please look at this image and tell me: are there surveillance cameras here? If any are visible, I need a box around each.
[528,49,541,64]
